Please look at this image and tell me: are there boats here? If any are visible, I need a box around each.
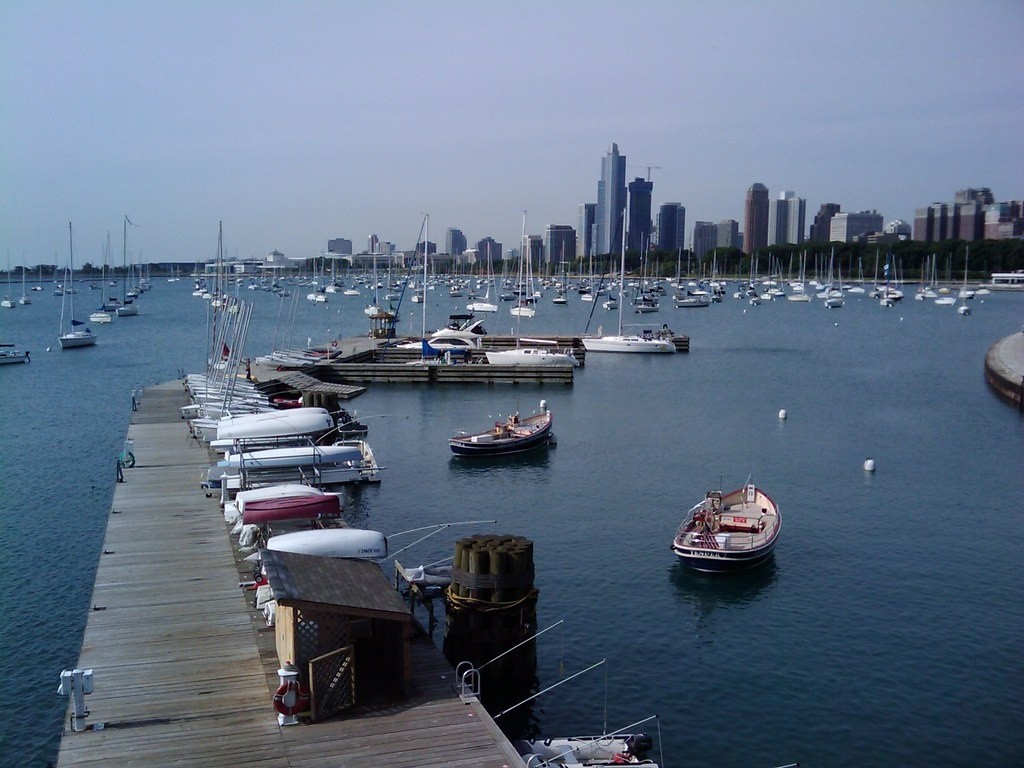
[979,269,1024,292]
[405,558,453,587]
[669,472,783,573]
[445,399,553,456]
[0,342,32,364]
[516,733,660,768]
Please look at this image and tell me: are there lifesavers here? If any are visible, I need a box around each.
[273,680,310,716]
[331,341,337,347]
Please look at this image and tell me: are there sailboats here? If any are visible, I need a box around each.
[483,209,582,370]
[55,221,98,348]
[1,208,992,628]
[581,208,677,355]
